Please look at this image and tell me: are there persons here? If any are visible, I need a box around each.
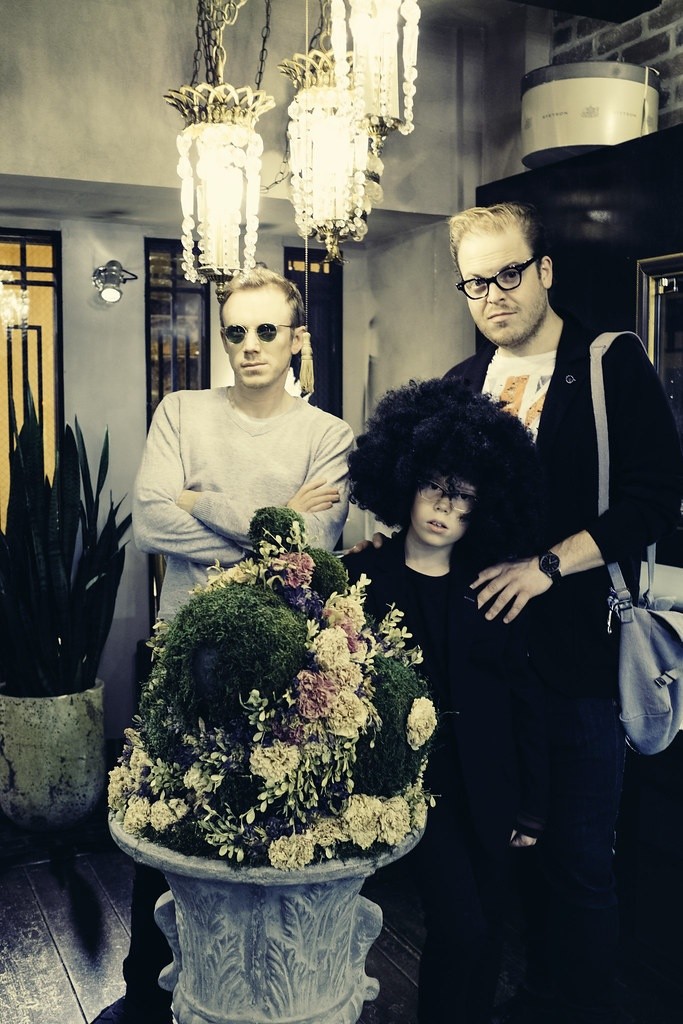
[89,264,359,1024]
[338,376,562,1024]
[350,203,683,1024]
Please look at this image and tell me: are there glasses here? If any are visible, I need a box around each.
[455,256,538,300]
[418,480,476,514]
[220,323,295,345]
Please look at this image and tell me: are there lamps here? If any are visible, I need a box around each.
[329,0,420,157]
[162,0,277,304]
[91,260,138,303]
[278,0,384,264]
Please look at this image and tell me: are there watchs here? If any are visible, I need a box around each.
[538,549,564,588]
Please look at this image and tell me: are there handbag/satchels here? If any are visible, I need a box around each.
[607,560,683,754]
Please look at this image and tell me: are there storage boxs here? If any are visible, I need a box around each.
[515,62,661,169]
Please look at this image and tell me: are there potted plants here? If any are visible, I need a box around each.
[0,376,132,835]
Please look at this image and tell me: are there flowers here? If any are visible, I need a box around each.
[106,505,442,873]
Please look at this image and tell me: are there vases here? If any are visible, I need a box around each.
[105,801,426,1024]
